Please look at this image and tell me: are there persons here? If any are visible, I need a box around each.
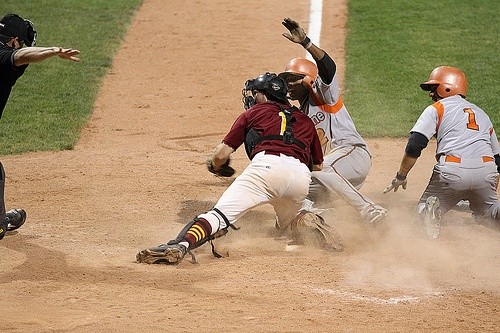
[0,14,80,238]
[382,67,500,241]
[135,72,346,264]
[274,18,388,232]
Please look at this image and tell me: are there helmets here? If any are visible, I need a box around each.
[278,57,317,86]
[420,65,467,99]
[243,72,289,109]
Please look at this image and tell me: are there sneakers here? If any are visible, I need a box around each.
[136,243,186,264]
[299,213,344,252]
[420,195,441,239]
[365,205,388,225]
[5,209,27,231]
